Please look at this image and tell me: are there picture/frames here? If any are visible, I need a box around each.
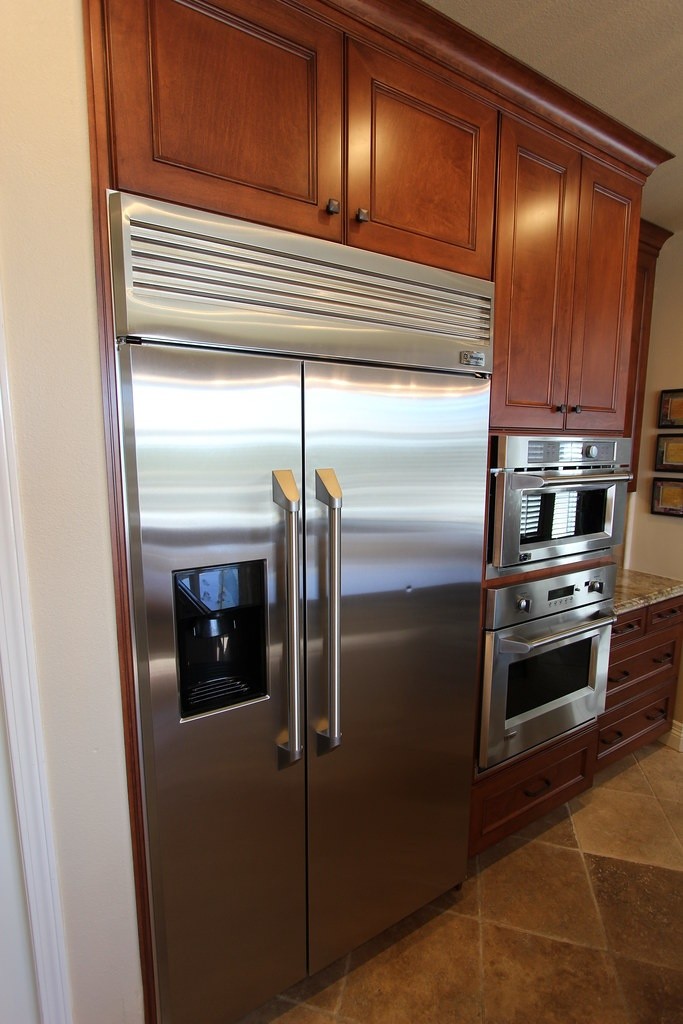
[657,389,683,429]
[650,477,683,517]
[654,434,683,474]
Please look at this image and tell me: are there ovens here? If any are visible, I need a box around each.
[476,560,619,769]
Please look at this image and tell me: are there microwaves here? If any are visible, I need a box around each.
[491,434,636,567]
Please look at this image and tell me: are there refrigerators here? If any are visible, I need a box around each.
[100,184,497,1024]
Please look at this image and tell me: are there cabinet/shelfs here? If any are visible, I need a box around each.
[84,1,500,285]
[469,713,596,858]
[593,570,683,775]
[496,36,682,438]
